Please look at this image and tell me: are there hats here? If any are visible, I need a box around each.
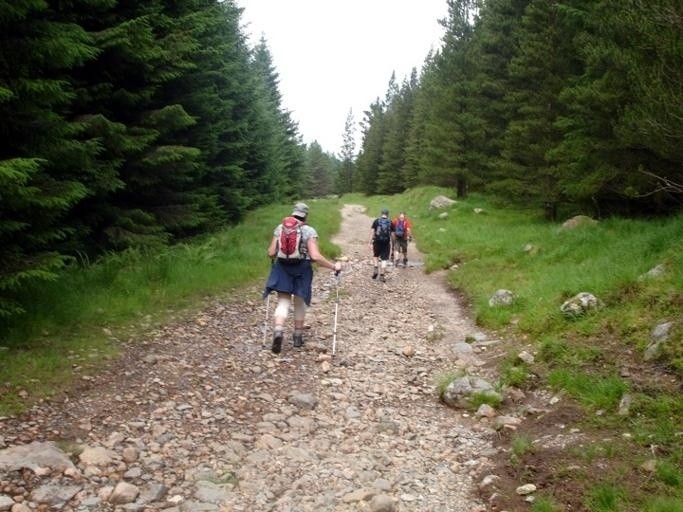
[289,202,309,218]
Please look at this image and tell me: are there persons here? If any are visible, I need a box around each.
[367,208,395,282]
[392,213,411,269]
[263,203,342,353]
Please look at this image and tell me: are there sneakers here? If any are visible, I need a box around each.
[402,258,409,266]
[379,272,385,282]
[293,334,309,347]
[371,266,378,280]
[271,330,283,355]
[394,257,400,266]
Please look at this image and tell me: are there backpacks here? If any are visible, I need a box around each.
[276,216,309,265]
[394,217,406,238]
[375,217,391,242]
